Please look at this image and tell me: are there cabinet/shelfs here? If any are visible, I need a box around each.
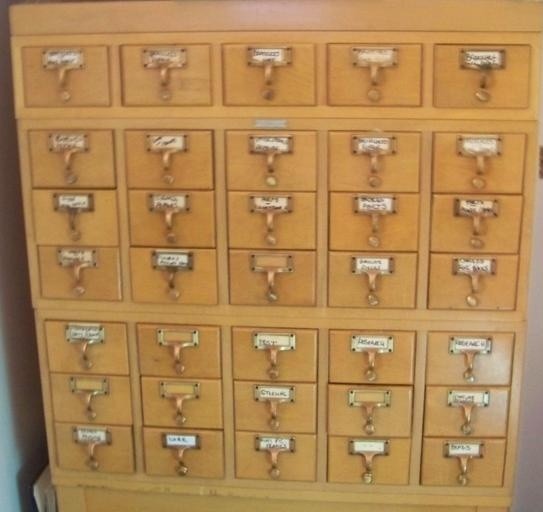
[9,0,542,512]
